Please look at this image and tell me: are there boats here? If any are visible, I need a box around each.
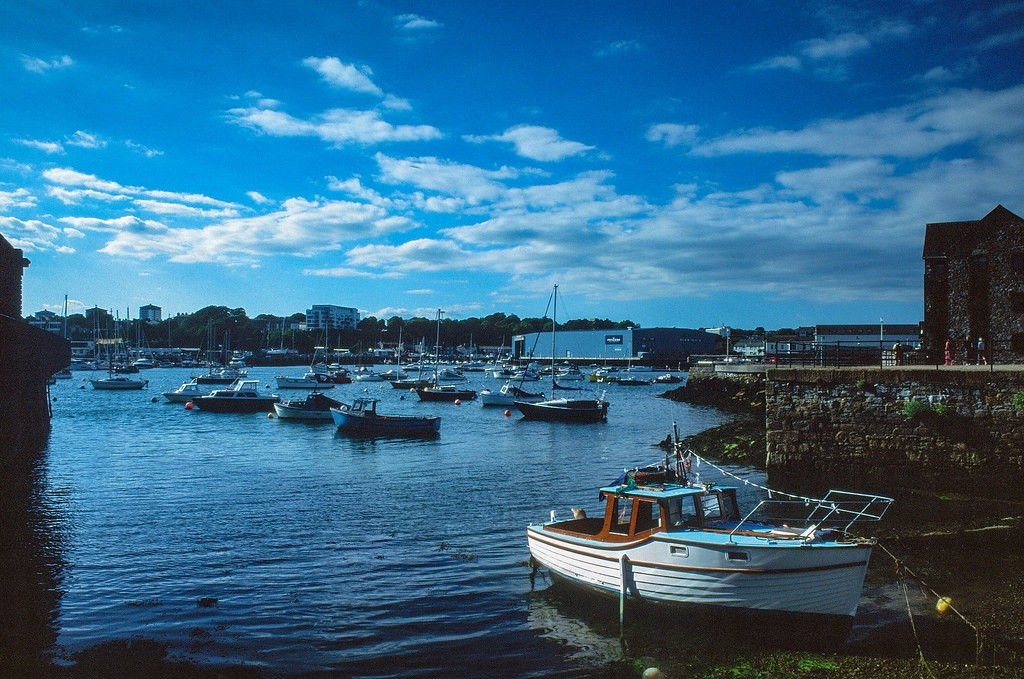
[380,369,408,380]
[588,371,624,382]
[274,391,352,421]
[159,353,223,368]
[162,378,208,403]
[618,378,653,385]
[437,368,467,380]
[275,374,335,388]
[493,360,584,381]
[191,378,279,412]
[655,373,681,383]
[329,397,442,434]
[481,385,545,405]
[353,367,384,382]
[526,421,894,644]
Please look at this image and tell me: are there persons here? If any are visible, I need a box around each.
[944,337,955,365]
[975,337,988,365]
[960,335,974,365]
[892,340,904,366]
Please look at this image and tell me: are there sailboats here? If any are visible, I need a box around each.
[416,308,478,401]
[401,335,436,371]
[456,332,485,371]
[90,304,158,374]
[304,323,352,383]
[51,295,72,379]
[90,308,149,390]
[188,317,249,384]
[513,283,610,422]
[390,335,432,389]
